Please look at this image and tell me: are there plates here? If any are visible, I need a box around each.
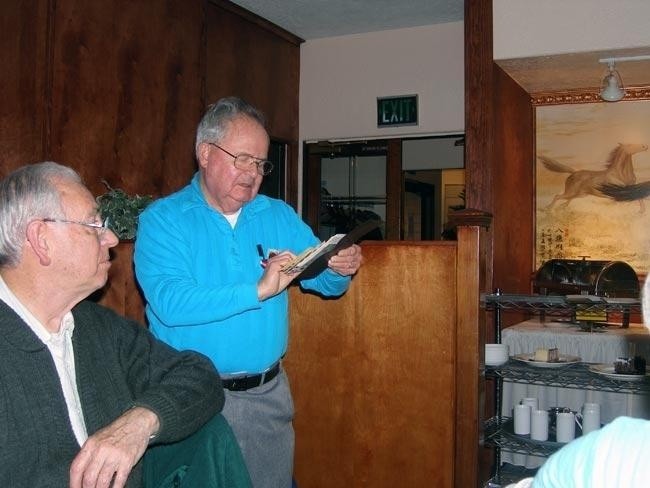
[586,363,650,381]
[485,342,509,366]
[509,352,582,368]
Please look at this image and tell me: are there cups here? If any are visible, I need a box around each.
[556,403,601,442]
[514,395,548,442]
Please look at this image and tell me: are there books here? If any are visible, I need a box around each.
[283,218,383,285]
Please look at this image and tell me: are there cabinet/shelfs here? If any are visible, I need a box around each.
[479,288,650,488]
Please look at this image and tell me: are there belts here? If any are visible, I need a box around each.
[222,364,279,391]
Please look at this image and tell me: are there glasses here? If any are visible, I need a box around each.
[209,142,274,176]
[44,217,109,233]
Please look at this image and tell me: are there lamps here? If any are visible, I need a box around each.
[599,58,625,102]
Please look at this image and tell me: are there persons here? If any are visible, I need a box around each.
[134,96,363,486]
[529,274,649,487]
[0,160,251,487]
